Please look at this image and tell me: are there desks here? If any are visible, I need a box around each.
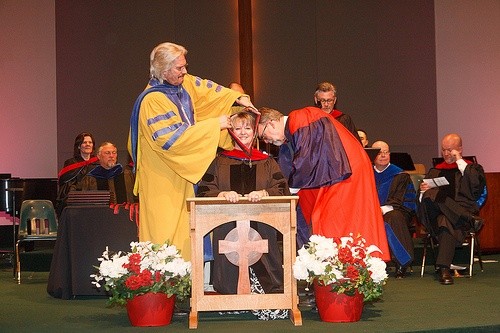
[405,171,500,252]
[46,202,139,301]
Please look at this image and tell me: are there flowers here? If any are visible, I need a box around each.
[89,238,193,308]
[292,232,388,301]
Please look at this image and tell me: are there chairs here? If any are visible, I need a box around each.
[13,199,58,285]
[420,215,486,278]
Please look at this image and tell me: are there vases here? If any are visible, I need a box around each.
[124,279,179,327]
[309,275,365,324]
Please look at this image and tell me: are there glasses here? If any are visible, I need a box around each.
[262,123,269,140]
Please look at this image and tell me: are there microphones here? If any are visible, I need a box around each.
[317,100,322,109]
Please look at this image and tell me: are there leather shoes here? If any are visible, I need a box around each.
[436,266,454,285]
[394,263,407,279]
[458,216,483,232]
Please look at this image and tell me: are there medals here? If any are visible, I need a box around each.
[248,158,252,168]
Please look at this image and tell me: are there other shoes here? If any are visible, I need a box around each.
[174,304,188,316]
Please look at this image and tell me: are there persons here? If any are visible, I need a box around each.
[371,141,417,279]
[313,82,363,146]
[195,110,291,295]
[128,42,261,316]
[414,134,486,285]
[55,131,139,222]
[257,107,391,308]
[356,130,369,148]
